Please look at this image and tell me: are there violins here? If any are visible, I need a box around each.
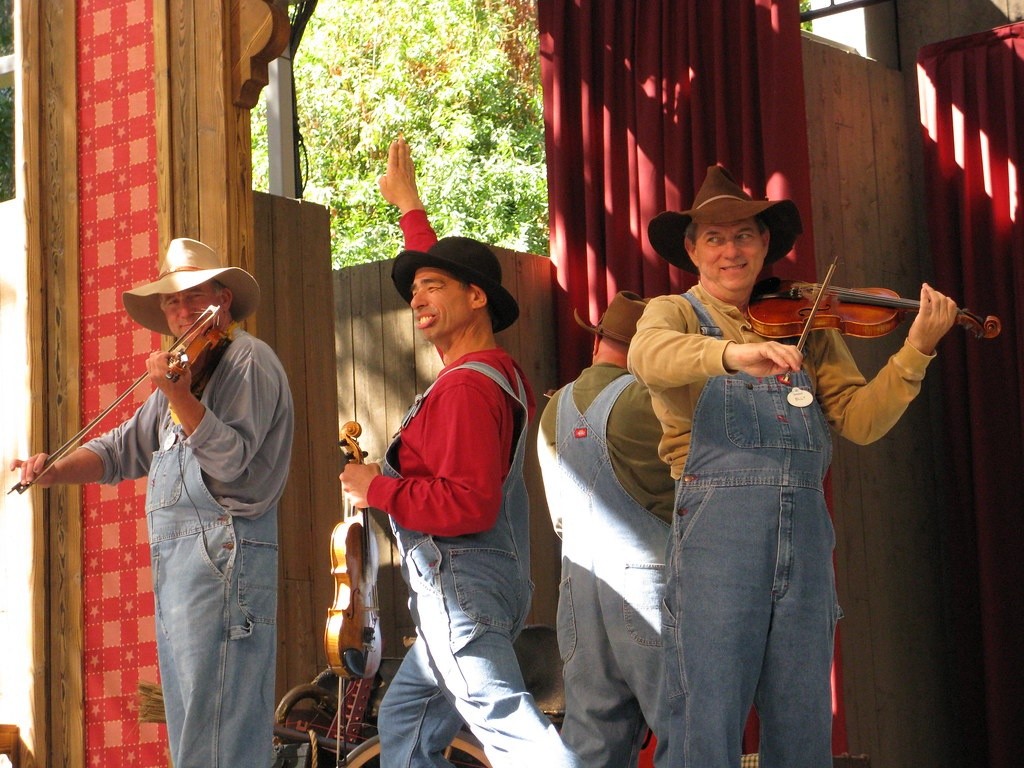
[162,324,232,397]
[746,275,1002,341]
[323,419,383,682]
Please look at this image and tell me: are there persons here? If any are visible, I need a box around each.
[537,290,684,768]
[9,238,295,768]
[338,140,591,768]
[626,166,958,768]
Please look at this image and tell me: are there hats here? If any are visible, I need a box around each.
[392,237,520,333]
[574,291,651,344]
[648,166,802,276]
[122,238,260,336]
